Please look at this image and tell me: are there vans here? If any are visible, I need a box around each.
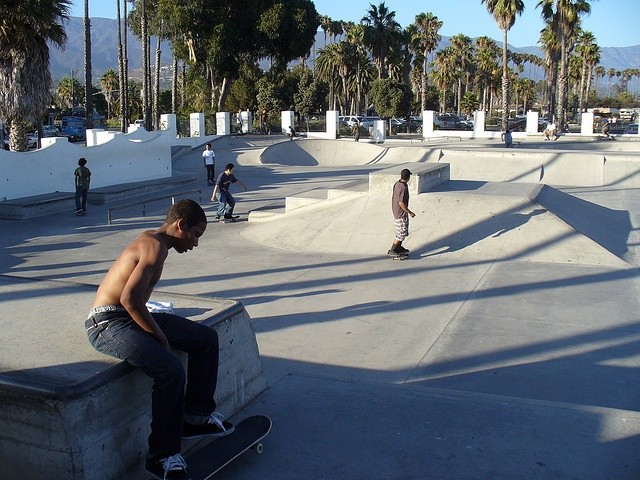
[339,115,370,130]
[366,117,388,127]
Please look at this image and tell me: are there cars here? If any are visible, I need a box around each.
[35,125,58,137]
[4,135,38,148]
[393,119,422,133]
[435,115,461,129]
[391,119,402,127]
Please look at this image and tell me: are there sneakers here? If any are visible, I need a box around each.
[387,249,408,257]
[75,208,84,213]
[211,179,216,183]
[215,216,220,219]
[207,180,213,184]
[224,215,232,219]
[144,451,192,480]
[398,246,409,253]
[182,411,235,440]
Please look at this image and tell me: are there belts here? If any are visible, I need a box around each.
[84,310,130,329]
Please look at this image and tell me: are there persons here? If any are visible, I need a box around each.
[201,143,217,183]
[392,168,416,255]
[237,110,244,134]
[85,199,235,479]
[600,115,610,138]
[501,128,516,148]
[289,126,297,141]
[74,158,92,213]
[211,163,248,220]
[351,123,360,141]
[543,120,557,142]
[260,109,268,135]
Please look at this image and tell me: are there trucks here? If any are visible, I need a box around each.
[61,116,108,139]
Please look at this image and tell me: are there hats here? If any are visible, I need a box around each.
[401,168,412,177]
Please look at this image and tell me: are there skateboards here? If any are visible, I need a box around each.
[388,250,409,260]
[217,215,240,223]
[74,212,87,216]
[183,414,272,480]
